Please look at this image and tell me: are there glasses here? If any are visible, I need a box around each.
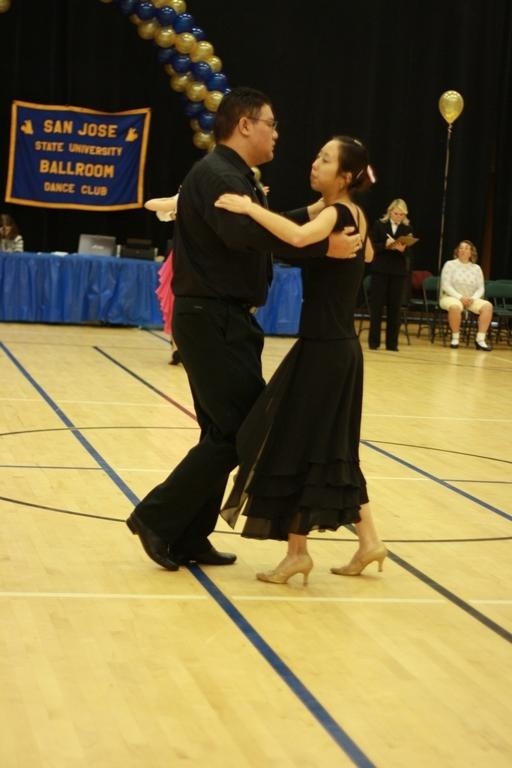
[237,114,279,131]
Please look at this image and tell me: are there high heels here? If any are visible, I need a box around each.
[331,543,388,575]
[451,338,459,349]
[257,556,314,585]
[475,339,492,350]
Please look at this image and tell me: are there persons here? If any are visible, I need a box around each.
[142,182,271,366]
[126,86,364,571]
[0,214,24,253]
[367,199,414,352]
[213,135,388,587]
[439,240,494,351]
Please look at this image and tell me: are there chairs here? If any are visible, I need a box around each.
[118,237,160,261]
[352,267,512,351]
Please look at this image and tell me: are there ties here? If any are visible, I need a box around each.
[250,174,273,288]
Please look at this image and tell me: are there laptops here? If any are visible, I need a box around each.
[78,234,116,256]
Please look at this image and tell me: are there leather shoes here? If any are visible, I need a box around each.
[169,352,180,365]
[169,546,237,565]
[126,510,180,571]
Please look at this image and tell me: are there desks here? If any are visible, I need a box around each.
[1,250,307,343]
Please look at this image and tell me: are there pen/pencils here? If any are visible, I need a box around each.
[387,234,395,242]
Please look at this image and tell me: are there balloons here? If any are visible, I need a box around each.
[100,0,232,154]
[438,89,465,129]
[0,0,11,14]
